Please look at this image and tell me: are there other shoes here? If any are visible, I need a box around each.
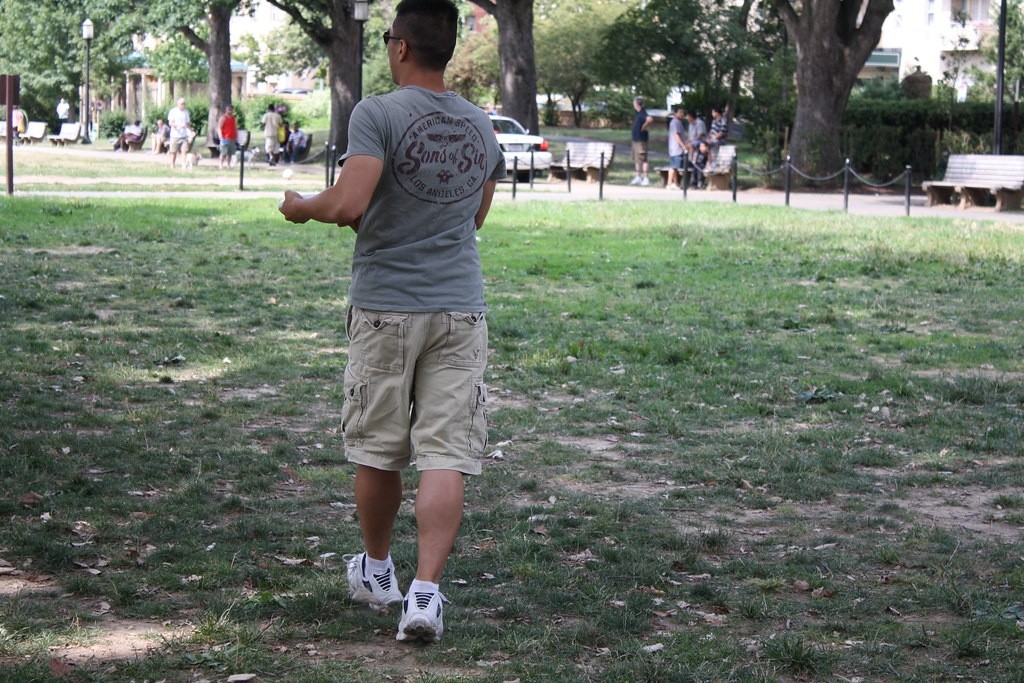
[630,176,651,186]
[667,181,681,190]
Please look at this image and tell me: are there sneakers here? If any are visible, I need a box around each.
[395,591,451,643]
[342,552,403,610]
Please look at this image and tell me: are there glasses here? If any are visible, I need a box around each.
[383,31,411,51]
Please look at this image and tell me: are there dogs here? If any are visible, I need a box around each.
[185,152,202,169]
[235,146,260,167]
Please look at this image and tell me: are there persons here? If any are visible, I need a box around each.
[629,98,654,186]
[705,106,728,146]
[148,120,170,154]
[280,0,507,637]
[284,121,307,163]
[168,98,190,169]
[56,98,70,134]
[115,119,142,152]
[690,142,711,188]
[685,109,708,161]
[666,105,688,190]
[273,120,292,163]
[218,105,237,169]
[260,104,283,166]
[13,105,24,135]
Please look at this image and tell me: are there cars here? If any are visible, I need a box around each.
[489,114,552,184]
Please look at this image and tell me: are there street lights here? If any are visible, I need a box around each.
[80,18,95,146]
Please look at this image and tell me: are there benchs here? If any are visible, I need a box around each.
[921,154,1024,212]
[124,126,149,152]
[47,122,82,149]
[18,121,48,145]
[266,134,312,164]
[546,142,616,184]
[0,120,6,143]
[653,144,739,191]
[207,130,252,159]
